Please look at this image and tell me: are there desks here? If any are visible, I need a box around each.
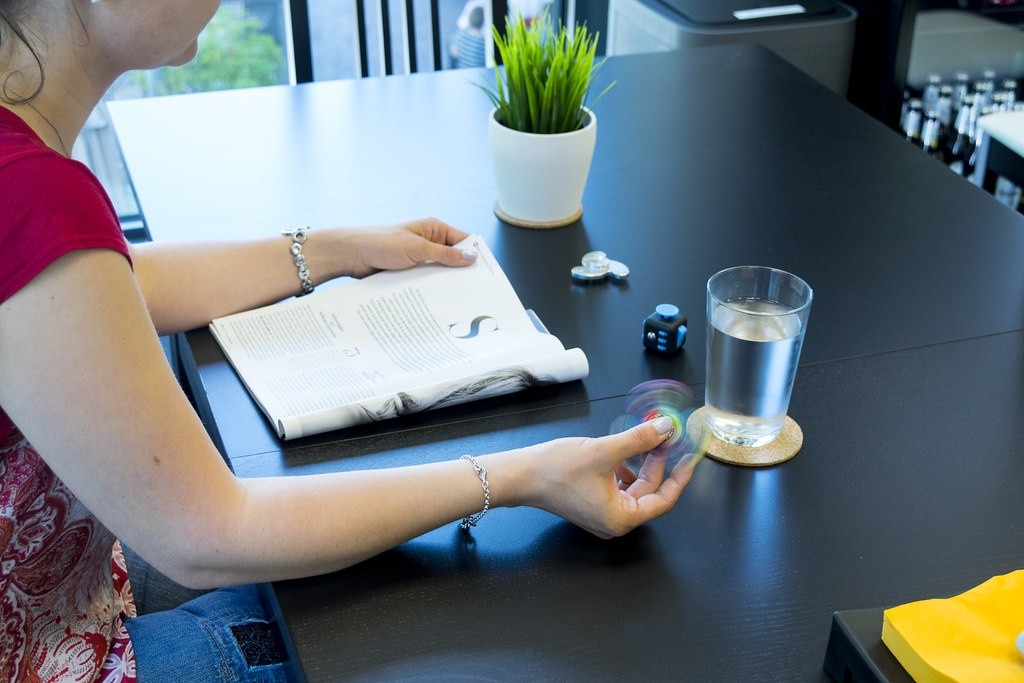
[102,41,1024,683]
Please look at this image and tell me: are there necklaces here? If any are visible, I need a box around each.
[0,83,71,159]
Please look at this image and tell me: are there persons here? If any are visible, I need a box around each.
[0,0,703,683]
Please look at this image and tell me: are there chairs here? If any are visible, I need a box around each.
[284,0,611,85]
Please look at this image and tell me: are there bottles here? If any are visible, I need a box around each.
[900,69,1024,184]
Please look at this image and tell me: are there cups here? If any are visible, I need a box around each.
[705,265,814,449]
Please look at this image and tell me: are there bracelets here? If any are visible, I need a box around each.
[458,455,490,529]
[279,225,314,299]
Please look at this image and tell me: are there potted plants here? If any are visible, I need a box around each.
[472,6,618,227]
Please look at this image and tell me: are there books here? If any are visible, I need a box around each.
[205,236,589,442]
